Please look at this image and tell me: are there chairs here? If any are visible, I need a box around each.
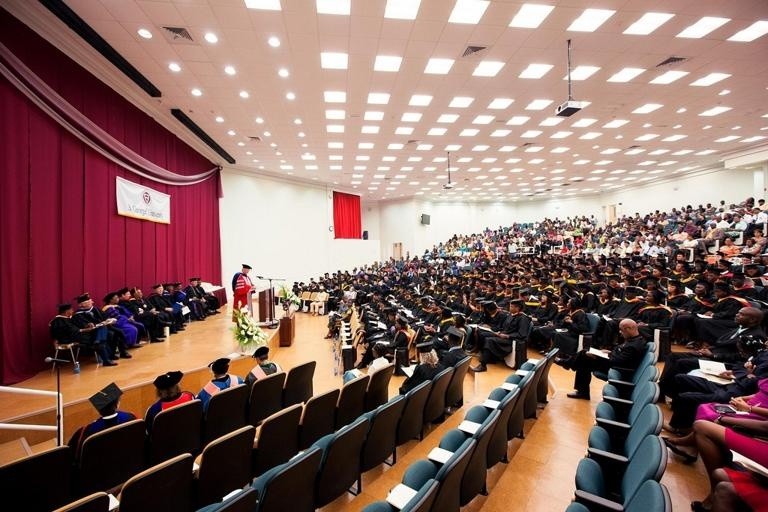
[46,276,228,375]
[570,329,768,512]
[2,347,561,512]
[258,215,768,376]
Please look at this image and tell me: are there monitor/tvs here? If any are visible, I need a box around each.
[422,214,430,225]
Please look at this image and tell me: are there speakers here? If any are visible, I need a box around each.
[363,231,368,239]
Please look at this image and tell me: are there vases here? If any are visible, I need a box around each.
[240,344,259,356]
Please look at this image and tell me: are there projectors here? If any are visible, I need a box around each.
[555,101,583,118]
[442,183,453,190]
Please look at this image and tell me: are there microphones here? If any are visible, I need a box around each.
[257,276,264,279]
[43,357,69,365]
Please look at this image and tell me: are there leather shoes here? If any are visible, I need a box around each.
[567,390,590,400]
[662,423,698,462]
[554,359,570,370]
[197,311,220,320]
[151,338,164,342]
[156,334,166,338]
[170,325,186,333]
[103,343,139,366]
[691,501,710,512]
[472,364,487,372]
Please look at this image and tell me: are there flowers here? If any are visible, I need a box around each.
[229,300,270,353]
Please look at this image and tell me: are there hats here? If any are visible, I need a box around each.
[242,264,252,269]
[397,316,408,327]
[252,347,269,358]
[88,382,123,415]
[430,249,768,313]
[58,288,128,312]
[152,277,201,289]
[208,359,230,374]
[416,341,433,353]
[446,325,463,342]
[153,371,183,390]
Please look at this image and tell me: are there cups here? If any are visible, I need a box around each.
[266,318,269,323]
[163,326,169,337]
